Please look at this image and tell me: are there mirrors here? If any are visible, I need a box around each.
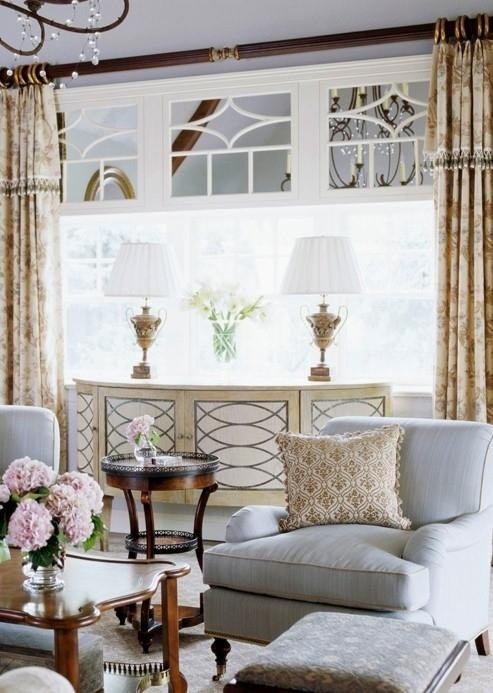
[165,83,299,201]
[56,98,144,205]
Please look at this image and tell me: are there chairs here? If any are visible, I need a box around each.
[0,402,62,476]
[200,414,492,682]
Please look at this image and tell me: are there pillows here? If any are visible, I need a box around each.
[270,427,415,534]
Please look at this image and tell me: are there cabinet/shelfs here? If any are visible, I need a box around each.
[70,377,389,508]
[98,450,219,653]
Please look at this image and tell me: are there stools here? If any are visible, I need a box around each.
[220,610,469,691]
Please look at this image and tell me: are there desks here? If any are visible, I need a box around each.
[0,553,193,692]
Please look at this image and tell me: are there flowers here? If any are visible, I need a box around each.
[1,455,106,569]
[181,270,270,359]
[123,412,159,451]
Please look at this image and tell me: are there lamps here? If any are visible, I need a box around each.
[280,235,350,380]
[278,78,430,191]
[104,241,182,380]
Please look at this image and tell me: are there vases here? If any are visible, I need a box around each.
[132,438,159,463]
[19,560,65,593]
[197,315,252,383]
